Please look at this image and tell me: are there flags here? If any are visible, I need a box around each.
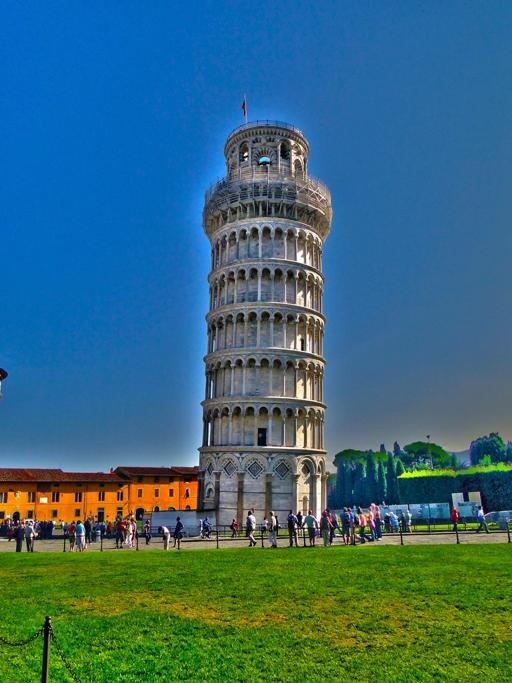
[242,100,246,115]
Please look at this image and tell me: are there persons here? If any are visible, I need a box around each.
[476,506,491,534]
[450,506,459,531]
